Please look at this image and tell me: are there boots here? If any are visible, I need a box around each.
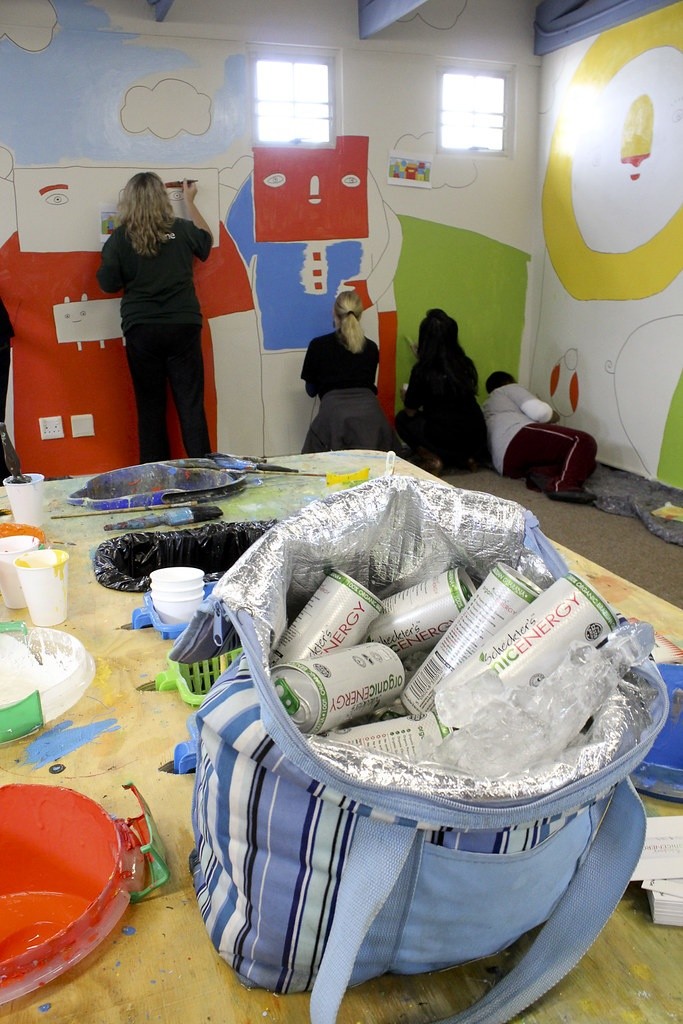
[418,446,443,476]
[464,456,478,473]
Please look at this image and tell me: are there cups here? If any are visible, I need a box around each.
[150,567,205,625]
[14,549,69,627]
[0,536,40,609]
[3,473,44,527]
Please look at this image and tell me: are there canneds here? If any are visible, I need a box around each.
[266,561,620,760]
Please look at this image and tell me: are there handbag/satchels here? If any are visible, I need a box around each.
[171,477,668,1024]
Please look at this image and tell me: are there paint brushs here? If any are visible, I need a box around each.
[49,500,197,519]
[179,179,199,185]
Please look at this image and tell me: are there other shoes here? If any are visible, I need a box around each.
[547,491,598,503]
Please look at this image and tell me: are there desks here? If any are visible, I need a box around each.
[0,448,683,1024]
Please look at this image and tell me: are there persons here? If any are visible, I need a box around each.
[482,371,599,503]
[394,309,489,476]
[95,171,214,464]
[0,298,16,486]
[300,291,403,456]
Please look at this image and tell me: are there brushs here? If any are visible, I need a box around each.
[214,458,299,476]
[204,453,268,463]
[104,506,224,532]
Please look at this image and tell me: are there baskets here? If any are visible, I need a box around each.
[134,582,247,703]
